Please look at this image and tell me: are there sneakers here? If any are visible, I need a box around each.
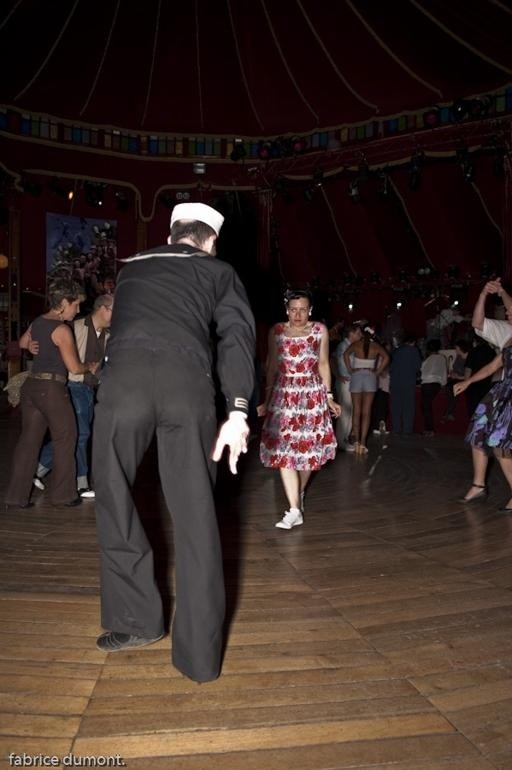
[374,430,389,434]
[78,488,95,498]
[33,475,46,490]
[275,510,305,530]
[338,443,355,451]
[380,422,386,434]
[300,491,306,513]
[96,631,165,652]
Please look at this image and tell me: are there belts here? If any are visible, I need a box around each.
[29,373,67,385]
[355,368,372,371]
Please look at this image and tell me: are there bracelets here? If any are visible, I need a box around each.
[499,287,505,298]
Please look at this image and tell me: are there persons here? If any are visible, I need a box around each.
[4,277,98,512]
[26,290,115,499]
[471,276,512,384]
[89,198,259,683]
[451,281,512,515]
[254,288,342,530]
[324,308,505,455]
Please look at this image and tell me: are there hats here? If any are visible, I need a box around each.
[171,202,226,238]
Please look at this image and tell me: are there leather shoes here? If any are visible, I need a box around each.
[6,502,36,509]
[65,499,82,507]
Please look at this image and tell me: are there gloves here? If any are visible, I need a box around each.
[213,410,251,475]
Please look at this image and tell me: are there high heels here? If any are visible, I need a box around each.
[448,482,488,506]
[354,442,360,452]
[360,445,368,454]
[497,506,512,514]
